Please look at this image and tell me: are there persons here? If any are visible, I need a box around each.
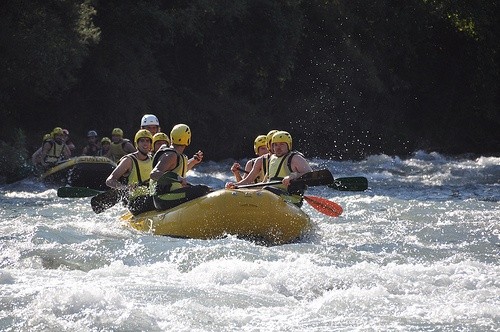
[225,129,314,209]
[32,115,214,216]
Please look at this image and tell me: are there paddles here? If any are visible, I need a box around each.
[328,177,368,192]
[157,171,193,187]
[58,187,102,198]
[237,168,343,217]
[91,178,150,214]
[235,168,334,189]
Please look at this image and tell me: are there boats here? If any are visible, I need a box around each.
[121,188,317,247]
[32,156,118,191]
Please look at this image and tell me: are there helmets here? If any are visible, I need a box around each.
[266,130,279,150]
[53,127,64,135]
[87,131,97,137]
[43,134,51,140]
[254,136,269,155]
[62,130,69,135]
[112,128,123,137]
[141,115,159,126]
[272,131,292,151]
[101,138,111,144]
[134,130,153,143]
[152,133,170,152]
[51,132,54,138]
[170,124,191,147]
